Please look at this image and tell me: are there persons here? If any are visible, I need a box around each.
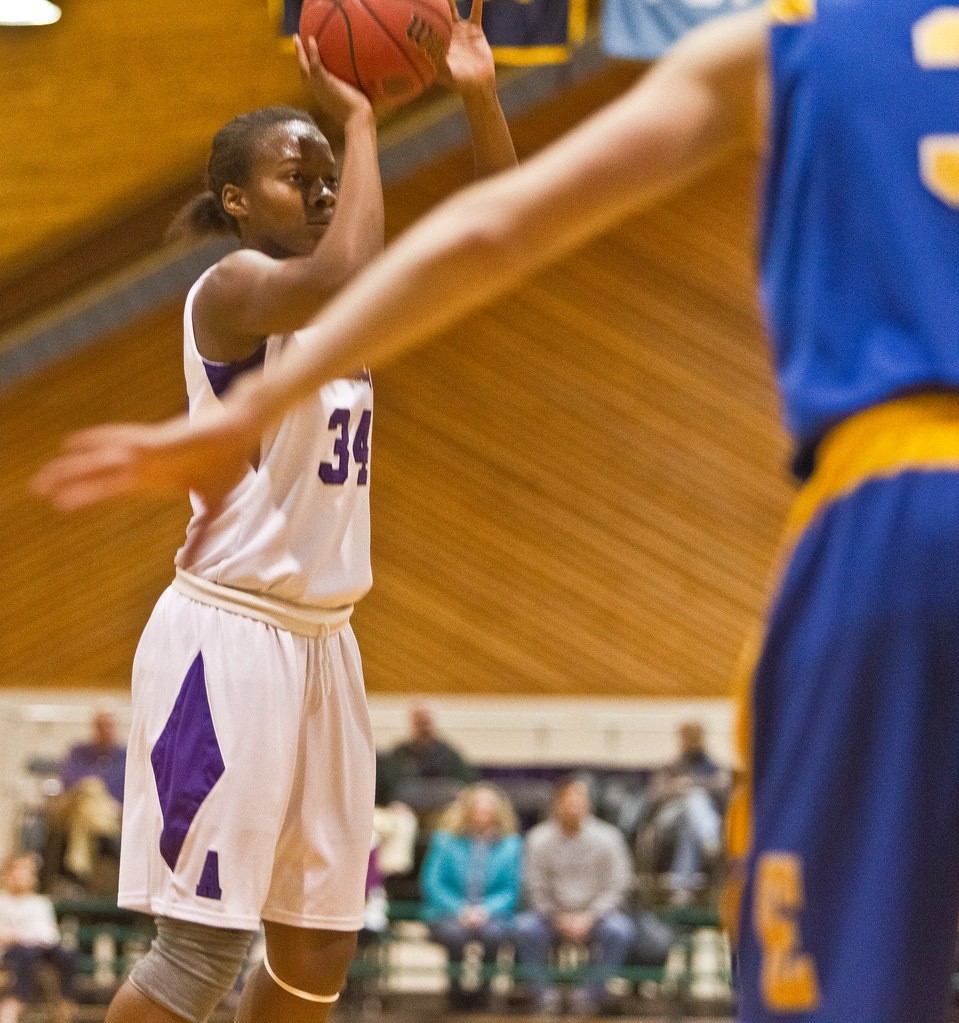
[384,711,468,833]
[36,0,959,1023]
[643,723,733,911]
[355,822,390,956]
[0,716,126,1023]
[101,0,521,1022]
[515,780,638,1013]
[418,783,522,1010]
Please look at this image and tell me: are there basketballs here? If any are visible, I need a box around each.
[297,0,454,111]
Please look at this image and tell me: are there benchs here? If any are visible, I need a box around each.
[9,755,730,1011]
